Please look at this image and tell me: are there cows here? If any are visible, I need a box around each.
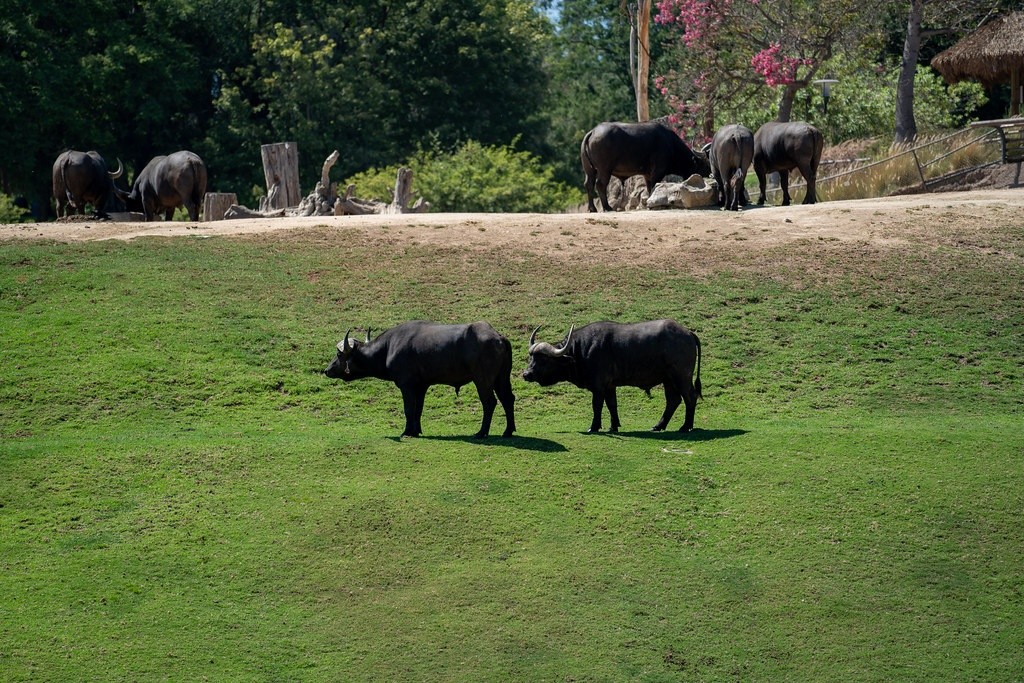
[749,121,823,205]
[582,120,695,214]
[523,318,703,433]
[112,150,207,223]
[690,124,755,211]
[52,150,123,219]
[324,319,517,439]
